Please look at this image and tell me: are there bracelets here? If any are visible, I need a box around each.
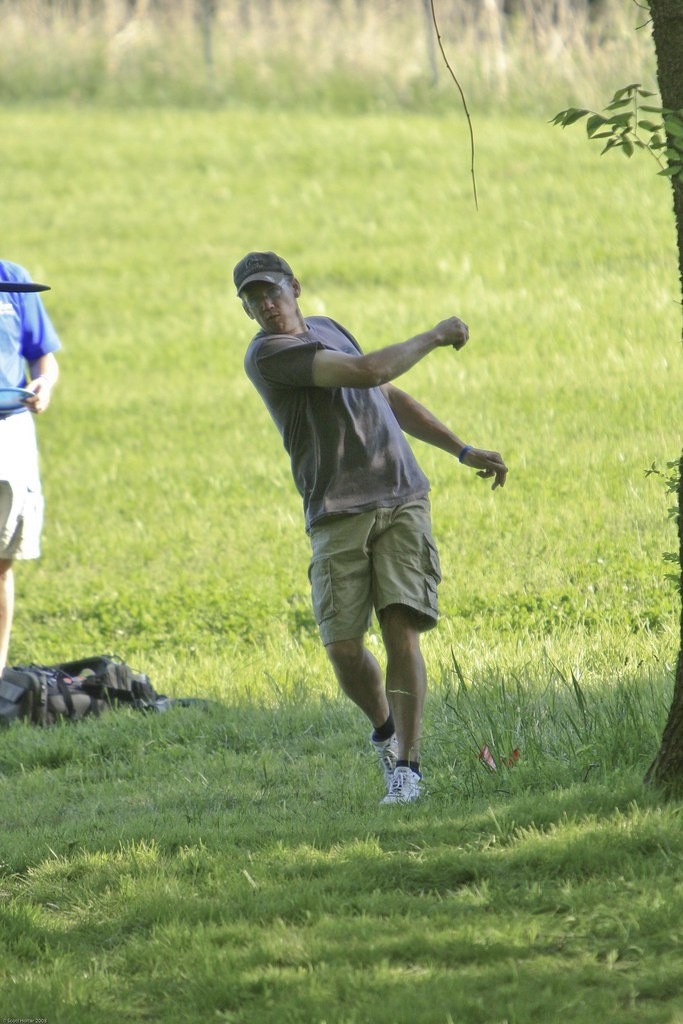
[458,445,472,462]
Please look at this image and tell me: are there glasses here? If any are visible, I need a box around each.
[246,278,288,308]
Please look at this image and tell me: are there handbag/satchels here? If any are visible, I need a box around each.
[0,655,167,727]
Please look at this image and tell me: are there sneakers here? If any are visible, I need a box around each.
[378,767,422,806]
[369,732,399,779]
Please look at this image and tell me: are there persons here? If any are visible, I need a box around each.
[0,258,61,685]
[232,251,508,804]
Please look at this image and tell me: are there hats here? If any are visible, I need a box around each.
[232,252,293,297]
